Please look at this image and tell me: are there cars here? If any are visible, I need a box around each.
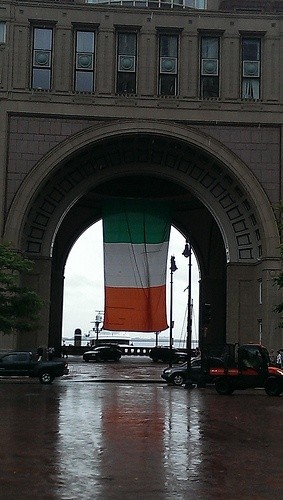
[161,355,225,387]
[149,346,188,364]
[83,345,122,362]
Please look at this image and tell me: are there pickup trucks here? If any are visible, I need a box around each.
[210,340,283,396]
[0,351,69,385]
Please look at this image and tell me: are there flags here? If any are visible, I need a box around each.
[102,210,169,331]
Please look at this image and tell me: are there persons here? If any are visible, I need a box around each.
[38,346,43,361]
[48,345,54,360]
[62,344,68,358]
[195,347,201,358]
[276,351,282,368]
[268,349,276,363]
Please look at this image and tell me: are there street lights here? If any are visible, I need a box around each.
[169,255,178,368]
[181,240,192,369]
[93,321,102,340]
